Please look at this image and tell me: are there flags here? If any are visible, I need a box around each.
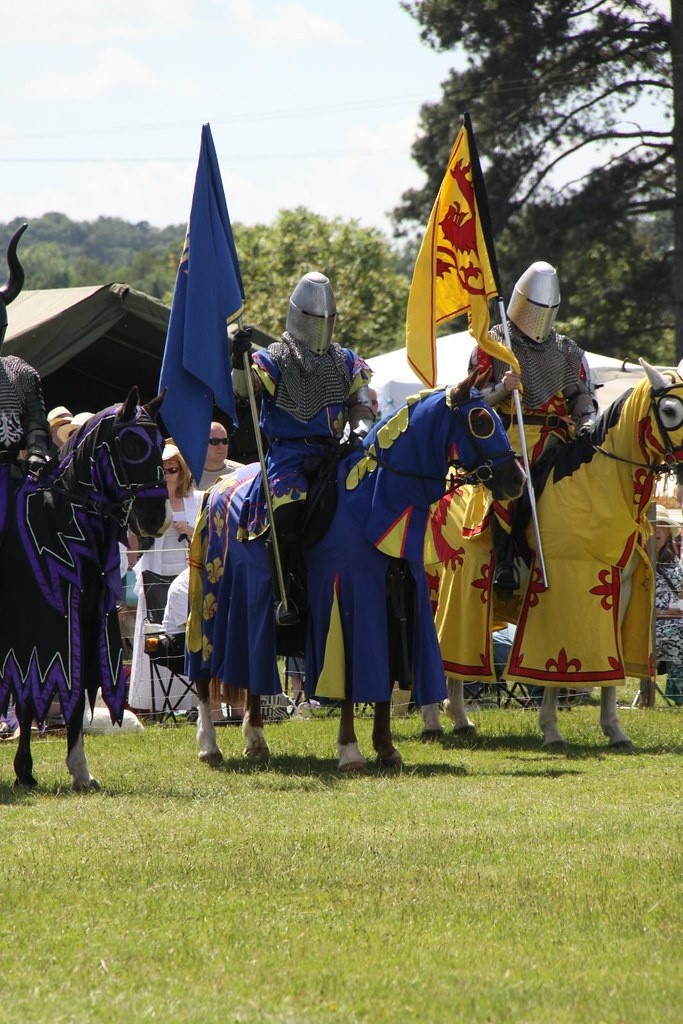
[159,122,248,486]
[404,126,499,390]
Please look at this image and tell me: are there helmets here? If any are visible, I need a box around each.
[285,271,336,356]
[506,261,561,343]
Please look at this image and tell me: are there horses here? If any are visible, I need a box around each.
[1,387,169,795]
[184,367,526,779]
[424,357,683,752]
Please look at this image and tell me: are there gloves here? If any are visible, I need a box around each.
[24,451,47,485]
[349,436,363,449]
[232,326,254,370]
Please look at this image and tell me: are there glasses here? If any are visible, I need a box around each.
[163,467,182,474]
[208,438,229,445]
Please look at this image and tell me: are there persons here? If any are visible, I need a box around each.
[46,405,321,725]
[491,620,579,708]
[231,268,376,627]
[0,292,53,549]
[651,502,683,707]
[467,258,599,601]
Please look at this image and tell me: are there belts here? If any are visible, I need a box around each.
[506,414,564,428]
[274,435,340,447]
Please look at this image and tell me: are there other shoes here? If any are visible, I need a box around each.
[493,552,514,601]
[273,596,303,625]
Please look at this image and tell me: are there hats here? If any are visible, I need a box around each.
[57,412,96,443]
[644,505,676,525]
[49,418,73,428]
[162,444,180,460]
[46,407,72,422]
